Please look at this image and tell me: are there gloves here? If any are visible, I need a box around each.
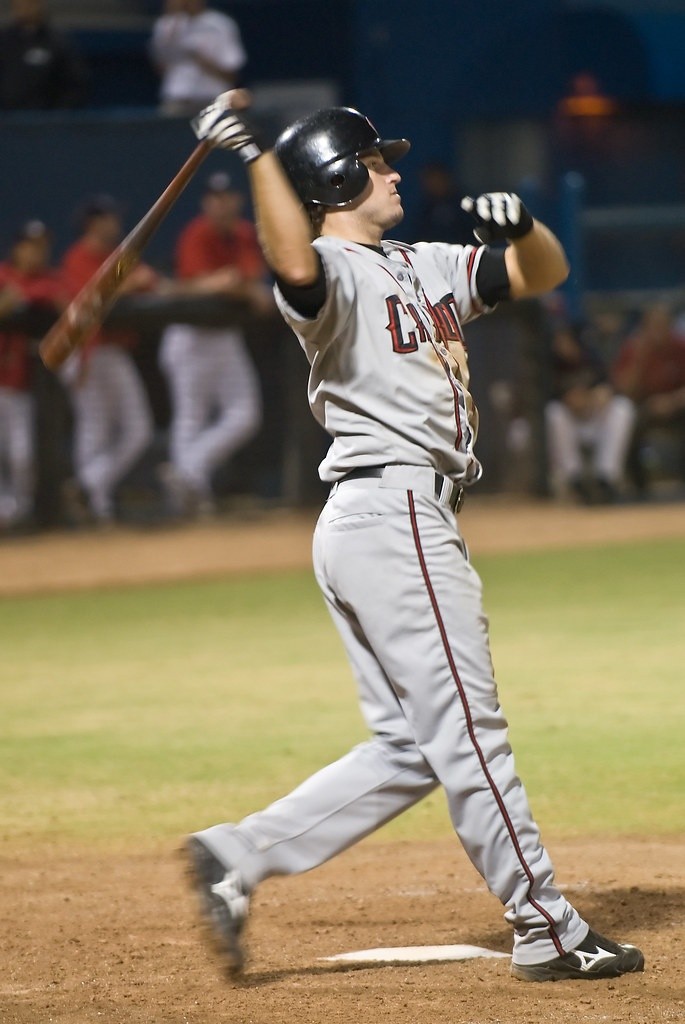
[191,104,270,164]
[461,190,534,242]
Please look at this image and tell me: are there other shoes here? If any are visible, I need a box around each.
[158,461,199,534]
[76,461,116,534]
[572,469,619,507]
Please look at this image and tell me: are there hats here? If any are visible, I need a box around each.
[81,196,128,217]
[198,171,240,197]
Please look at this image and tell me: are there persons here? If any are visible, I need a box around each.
[184,89,644,985]
[0,0,685,532]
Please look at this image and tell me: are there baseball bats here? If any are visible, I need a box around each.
[36,87,250,371]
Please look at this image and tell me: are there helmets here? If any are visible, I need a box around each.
[271,107,411,207]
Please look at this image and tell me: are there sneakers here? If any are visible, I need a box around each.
[175,835,255,979]
[511,928,645,983]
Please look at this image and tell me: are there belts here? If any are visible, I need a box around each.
[338,463,468,514]
